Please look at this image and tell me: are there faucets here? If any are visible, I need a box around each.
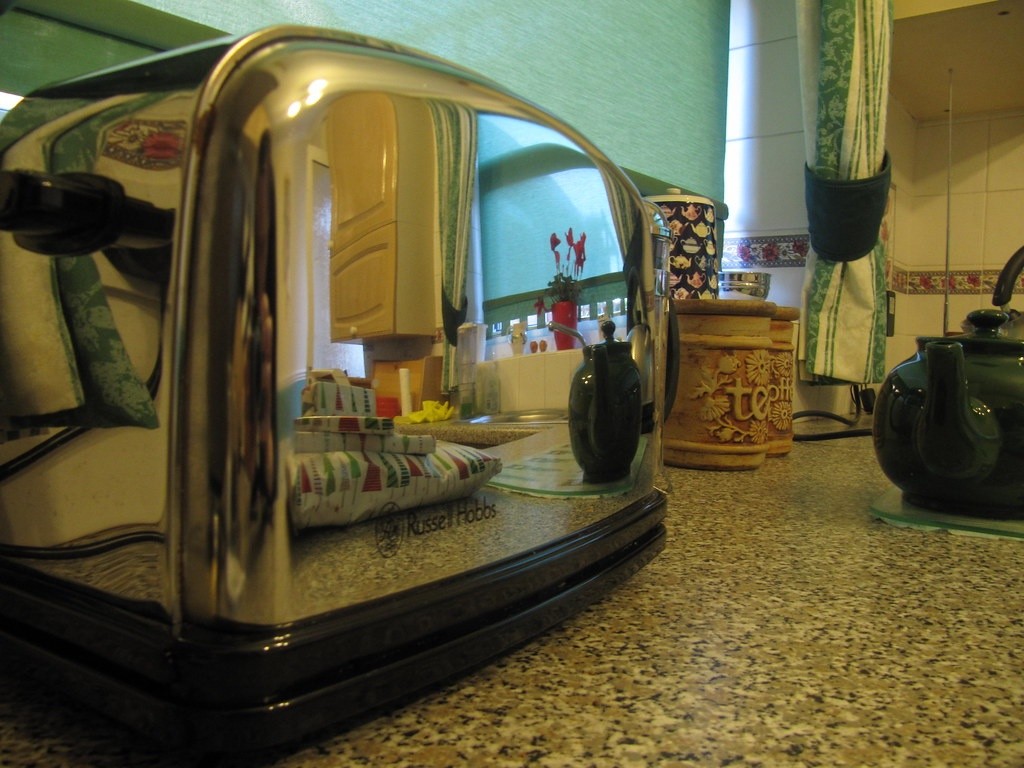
[546,320,588,347]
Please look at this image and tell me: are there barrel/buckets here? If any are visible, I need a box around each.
[637,187,719,300]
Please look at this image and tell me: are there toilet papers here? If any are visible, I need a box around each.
[398,368,413,416]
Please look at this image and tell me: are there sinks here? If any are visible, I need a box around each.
[469,408,570,425]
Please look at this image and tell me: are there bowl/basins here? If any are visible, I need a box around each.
[717,272,772,301]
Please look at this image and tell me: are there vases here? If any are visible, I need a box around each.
[551,300,576,350]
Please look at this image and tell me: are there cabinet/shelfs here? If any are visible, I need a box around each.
[327,91,435,344]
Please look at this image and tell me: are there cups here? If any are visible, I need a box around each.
[506,321,528,356]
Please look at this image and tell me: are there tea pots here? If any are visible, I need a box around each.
[873,245,1024,523]
[567,319,644,486]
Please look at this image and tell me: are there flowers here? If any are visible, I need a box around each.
[533,227,586,316]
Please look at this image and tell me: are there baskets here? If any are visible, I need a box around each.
[376,397,401,419]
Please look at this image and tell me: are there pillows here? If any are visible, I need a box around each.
[0,89,200,429]
[293,440,502,528]
